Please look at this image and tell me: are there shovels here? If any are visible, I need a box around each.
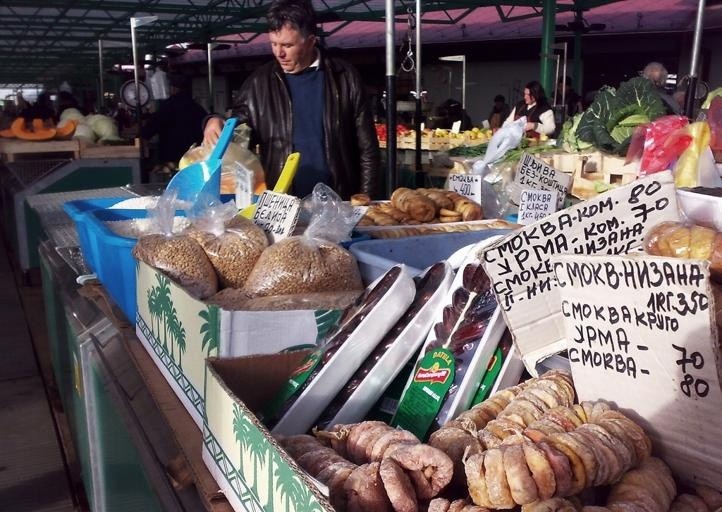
[237,152,301,220]
[166,118,239,217]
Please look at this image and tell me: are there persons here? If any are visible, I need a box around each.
[156,72,210,164]
[428,77,584,146]
[202,0,381,203]
[0,88,152,131]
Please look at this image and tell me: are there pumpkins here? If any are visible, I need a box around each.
[0,117,76,140]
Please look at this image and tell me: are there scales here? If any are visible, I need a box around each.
[120,79,150,134]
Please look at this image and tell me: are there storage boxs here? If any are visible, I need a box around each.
[457,149,629,222]
[201,348,331,507]
[134,258,352,430]
[349,227,513,286]
[87,212,190,330]
[677,180,722,234]
[62,199,141,276]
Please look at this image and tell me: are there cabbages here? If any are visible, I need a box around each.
[58,107,121,141]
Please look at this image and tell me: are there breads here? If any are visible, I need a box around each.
[351,187,520,240]
